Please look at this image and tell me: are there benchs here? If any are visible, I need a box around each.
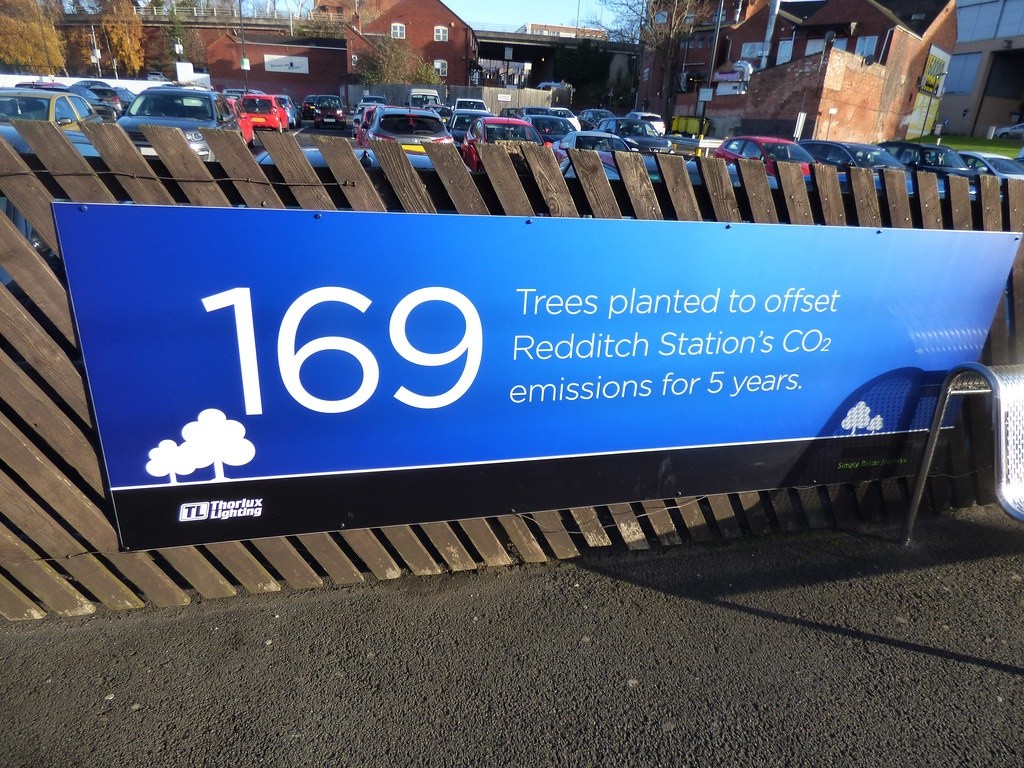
[899,359,1024,546]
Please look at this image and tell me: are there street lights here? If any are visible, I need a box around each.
[918,71,948,142]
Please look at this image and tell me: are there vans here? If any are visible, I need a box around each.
[404,89,442,110]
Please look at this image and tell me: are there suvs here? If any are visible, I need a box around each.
[147,72,169,82]
[115,81,244,162]
[626,112,666,137]
[592,118,676,153]
[367,104,454,171]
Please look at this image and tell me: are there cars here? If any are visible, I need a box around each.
[15,82,66,89]
[445,110,496,150]
[523,115,577,149]
[560,147,778,198]
[0,88,103,131]
[713,136,824,176]
[551,130,632,167]
[301,95,317,120]
[279,95,302,129]
[0,126,134,368]
[227,99,256,149]
[222,88,266,95]
[451,98,491,113]
[500,107,554,124]
[314,95,348,130]
[420,104,454,128]
[85,86,139,117]
[241,94,289,134]
[253,143,476,215]
[957,150,1024,204]
[798,139,977,202]
[35,86,118,124]
[71,80,110,88]
[875,142,1003,204]
[550,107,581,132]
[995,122,1024,139]
[361,96,388,105]
[577,109,616,131]
[353,106,400,148]
[352,103,387,138]
[222,93,242,100]
[461,117,553,172]
